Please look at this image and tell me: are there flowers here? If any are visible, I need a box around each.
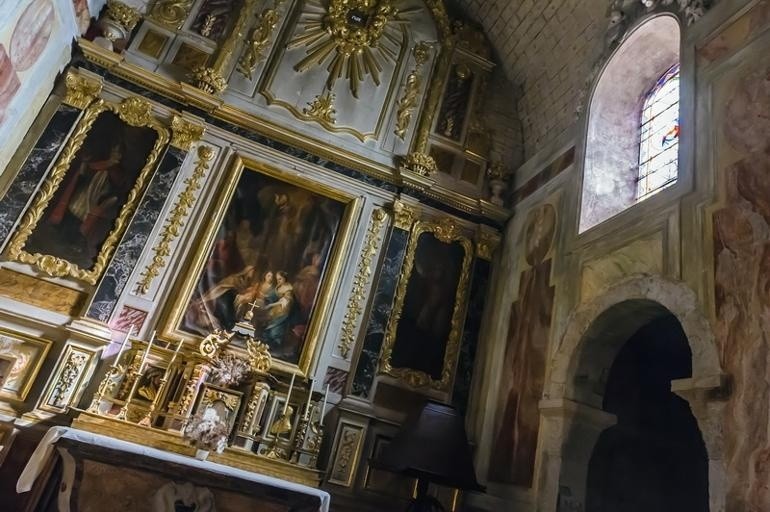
[104,0,142,33]
[405,150,438,173]
[485,159,514,181]
[184,60,229,93]
[182,408,230,455]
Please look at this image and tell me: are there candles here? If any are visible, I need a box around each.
[282,372,330,426]
[109,324,186,383]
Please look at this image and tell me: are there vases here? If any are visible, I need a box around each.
[91,22,127,53]
[197,79,215,94]
[489,182,505,208]
[411,163,428,176]
[195,449,211,461]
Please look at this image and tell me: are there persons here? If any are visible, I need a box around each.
[185,216,327,366]
[269,405,294,437]
[135,367,163,401]
[47,135,140,233]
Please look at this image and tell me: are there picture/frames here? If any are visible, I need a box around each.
[424,136,488,199]
[375,217,475,395]
[157,151,362,380]
[0,96,170,285]
[127,19,215,85]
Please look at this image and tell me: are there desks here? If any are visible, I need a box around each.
[21,425,330,512]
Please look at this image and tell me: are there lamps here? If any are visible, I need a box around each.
[367,403,488,512]
